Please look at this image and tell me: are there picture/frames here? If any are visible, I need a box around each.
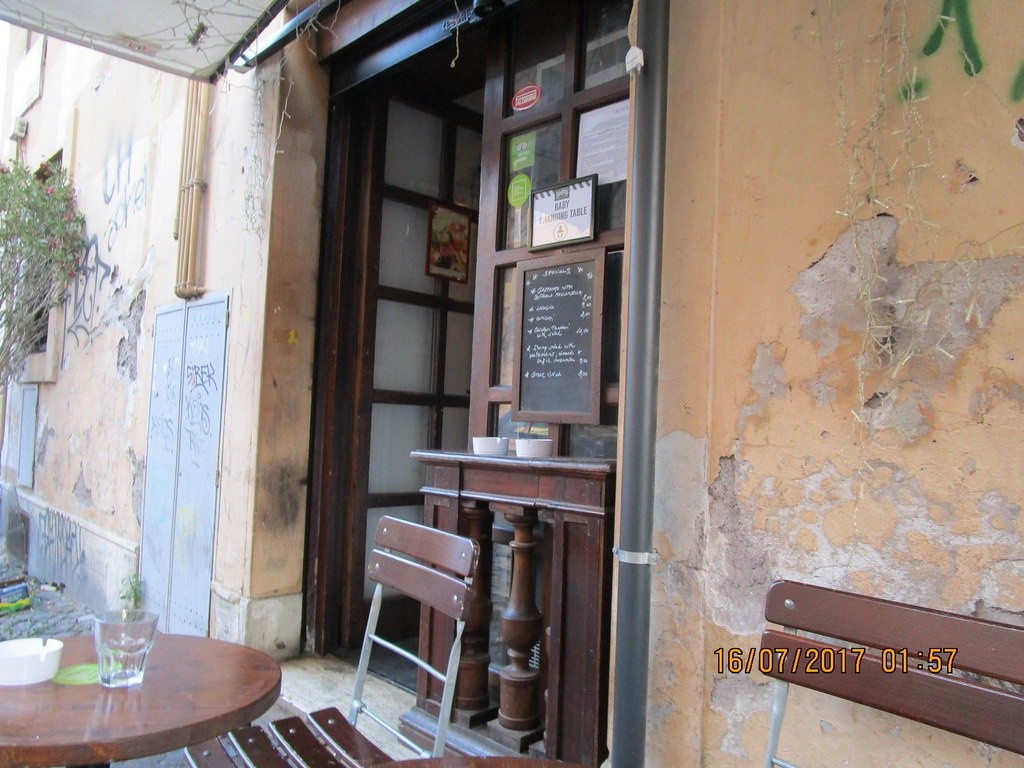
[511,249,605,425]
[526,173,598,252]
[425,201,473,284]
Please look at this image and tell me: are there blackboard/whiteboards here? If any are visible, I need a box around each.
[510,247,604,426]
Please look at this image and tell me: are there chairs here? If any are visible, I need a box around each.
[759,580,1024,768]
[182,516,481,768]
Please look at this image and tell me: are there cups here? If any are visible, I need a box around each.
[94,609,159,688]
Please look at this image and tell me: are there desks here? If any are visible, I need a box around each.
[398,448,617,768]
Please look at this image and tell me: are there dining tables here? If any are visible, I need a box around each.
[1,634,282,768]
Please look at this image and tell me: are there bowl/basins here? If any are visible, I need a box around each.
[515,439,553,458]
[0,637,64,687]
[472,437,508,457]
